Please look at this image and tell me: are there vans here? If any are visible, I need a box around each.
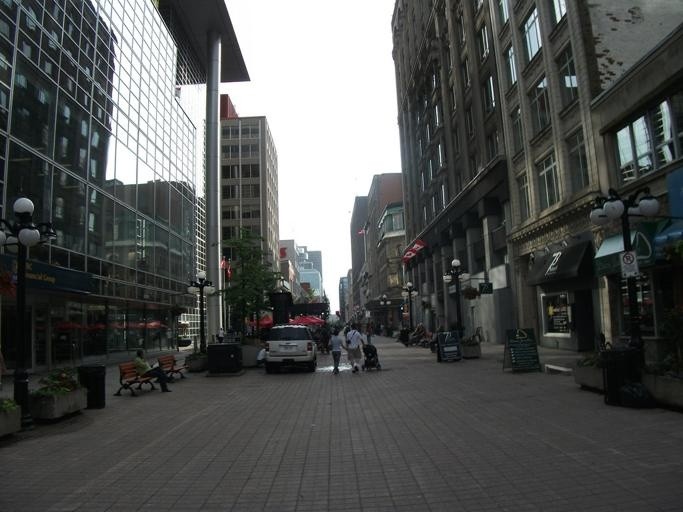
[263,323,320,374]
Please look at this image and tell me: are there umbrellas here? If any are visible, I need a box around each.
[58,319,167,339]
[291,316,323,331]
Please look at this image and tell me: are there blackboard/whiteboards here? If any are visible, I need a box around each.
[507,327,541,370]
[438,331,462,360]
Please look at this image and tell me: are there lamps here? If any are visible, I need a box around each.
[544,240,553,256]
[529,245,537,259]
[561,232,572,249]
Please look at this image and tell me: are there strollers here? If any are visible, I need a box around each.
[361,344,382,371]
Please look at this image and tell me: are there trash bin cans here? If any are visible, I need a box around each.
[79,364,105,409]
[600,347,643,406]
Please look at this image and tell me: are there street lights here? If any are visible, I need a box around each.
[141,293,150,353]
[179,321,189,336]
[442,257,472,337]
[0,196,59,411]
[345,305,365,326]
[380,294,392,336]
[400,282,419,328]
[584,185,663,344]
[185,270,217,352]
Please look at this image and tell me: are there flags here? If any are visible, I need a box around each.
[412,239,425,253]
[221,259,226,269]
[227,264,232,279]
[403,248,417,264]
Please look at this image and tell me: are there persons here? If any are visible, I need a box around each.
[133,349,175,392]
[218,328,224,343]
[319,321,374,374]
[408,323,425,346]
[256,347,269,367]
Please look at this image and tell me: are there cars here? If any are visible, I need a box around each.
[168,334,193,347]
